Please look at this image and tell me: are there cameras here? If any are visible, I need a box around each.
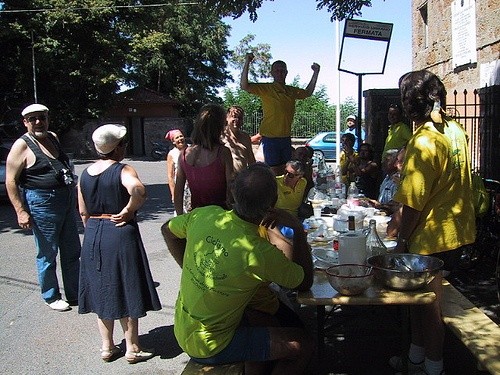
[55,169,74,186]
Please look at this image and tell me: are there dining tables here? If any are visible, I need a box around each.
[282,192,436,375]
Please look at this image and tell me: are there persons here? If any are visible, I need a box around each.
[165,128,188,217]
[215,104,254,215]
[397,71,476,291]
[343,115,366,150]
[342,143,378,200]
[303,145,315,190]
[289,147,309,186]
[161,162,330,374]
[269,160,307,224]
[74,121,164,364]
[470,171,489,230]
[375,149,396,209]
[175,105,234,220]
[338,135,359,187]
[237,52,320,175]
[382,147,405,215]
[383,102,412,171]
[7,103,85,311]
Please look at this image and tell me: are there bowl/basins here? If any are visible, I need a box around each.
[367,252,445,291]
[325,263,377,297]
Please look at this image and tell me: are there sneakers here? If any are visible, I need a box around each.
[43,296,71,312]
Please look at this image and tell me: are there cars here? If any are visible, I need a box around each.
[0,143,12,200]
[306,130,358,162]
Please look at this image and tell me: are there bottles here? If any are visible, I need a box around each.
[335,165,343,182]
[347,182,359,205]
[338,216,367,268]
[326,165,335,182]
[341,184,346,196]
[365,219,387,260]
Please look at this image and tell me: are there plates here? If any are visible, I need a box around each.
[307,230,340,242]
[311,248,338,265]
[313,259,330,270]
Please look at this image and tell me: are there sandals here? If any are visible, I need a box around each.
[125,350,154,364]
[100,346,123,362]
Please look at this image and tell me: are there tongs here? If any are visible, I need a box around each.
[365,266,373,277]
[394,257,411,271]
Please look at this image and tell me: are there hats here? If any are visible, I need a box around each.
[22,104,49,116]
[346,114,357,120]
[92,123,128,153]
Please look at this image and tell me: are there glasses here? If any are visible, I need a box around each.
[120,138,128,144]
[24,114,47,121]
[284,169,301,178]
